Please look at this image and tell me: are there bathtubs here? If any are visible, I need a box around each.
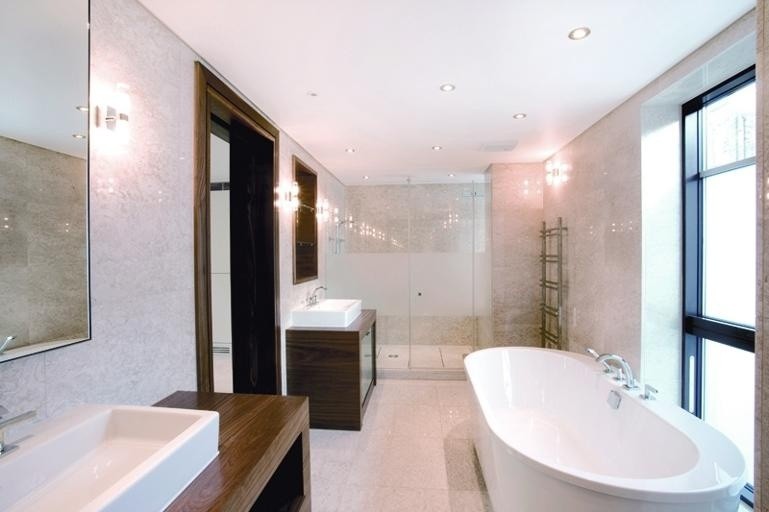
[464,346,748,512]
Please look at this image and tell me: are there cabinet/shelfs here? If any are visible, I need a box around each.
[223,397,311,512]
[286,310,377,430]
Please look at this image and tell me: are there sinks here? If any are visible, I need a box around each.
[292,299,361,327]
[0,408,219,512]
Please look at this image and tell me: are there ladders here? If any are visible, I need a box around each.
[539,217,568,350]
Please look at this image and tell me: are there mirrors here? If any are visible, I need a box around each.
[0,0,91,363]
[292,154,319,285]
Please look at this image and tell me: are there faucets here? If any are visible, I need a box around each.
[585,348,610,372]
[597,353,634,387]
[0,336,17,355]
[310,285,327,300]
[0,404,36,456]
